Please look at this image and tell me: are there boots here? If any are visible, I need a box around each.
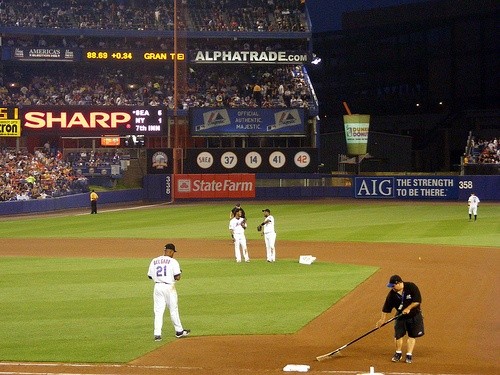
[469,214,472,220]
[474,215,477,220]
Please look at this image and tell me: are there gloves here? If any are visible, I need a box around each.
[256,225,262,231]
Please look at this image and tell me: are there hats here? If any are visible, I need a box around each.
[164,243,176,252]
[386,275,403,287]
[262,209,270,213]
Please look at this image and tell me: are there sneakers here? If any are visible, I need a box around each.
[175,330,190,338]
[405,355,412,363]
[154,336,162,342]
[391,353,402,362]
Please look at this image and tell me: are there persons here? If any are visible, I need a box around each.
[463,134,500,166]
[89,189,99,214]
[467,192,481,220]
[257,209,277,262]
[146,243,191,341]
[0,0,317,201]
[376,275,425,364]
[228,202,252,263]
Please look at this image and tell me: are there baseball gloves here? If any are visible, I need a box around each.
[257,225,262,231]
